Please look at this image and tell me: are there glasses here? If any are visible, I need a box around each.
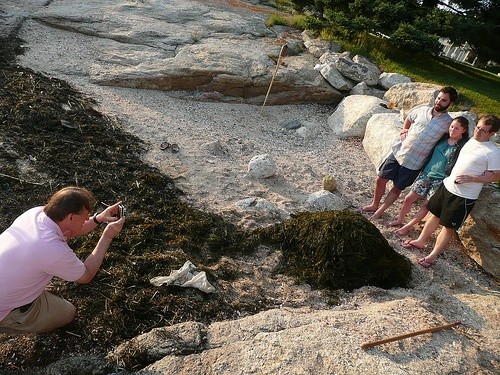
[474,124,490,135]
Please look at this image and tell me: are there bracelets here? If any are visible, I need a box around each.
[94,212,104,225]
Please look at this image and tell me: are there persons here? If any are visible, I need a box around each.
[1,186,127,334]
[399,114,500,268]
[389,115,494,240]
[361,85,457,221]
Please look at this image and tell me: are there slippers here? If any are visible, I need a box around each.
[401,238,425,249]
[418,256,437,267]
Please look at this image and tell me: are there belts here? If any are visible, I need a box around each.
[10,296,36,313]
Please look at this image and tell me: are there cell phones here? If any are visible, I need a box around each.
[117,205,124,220]
